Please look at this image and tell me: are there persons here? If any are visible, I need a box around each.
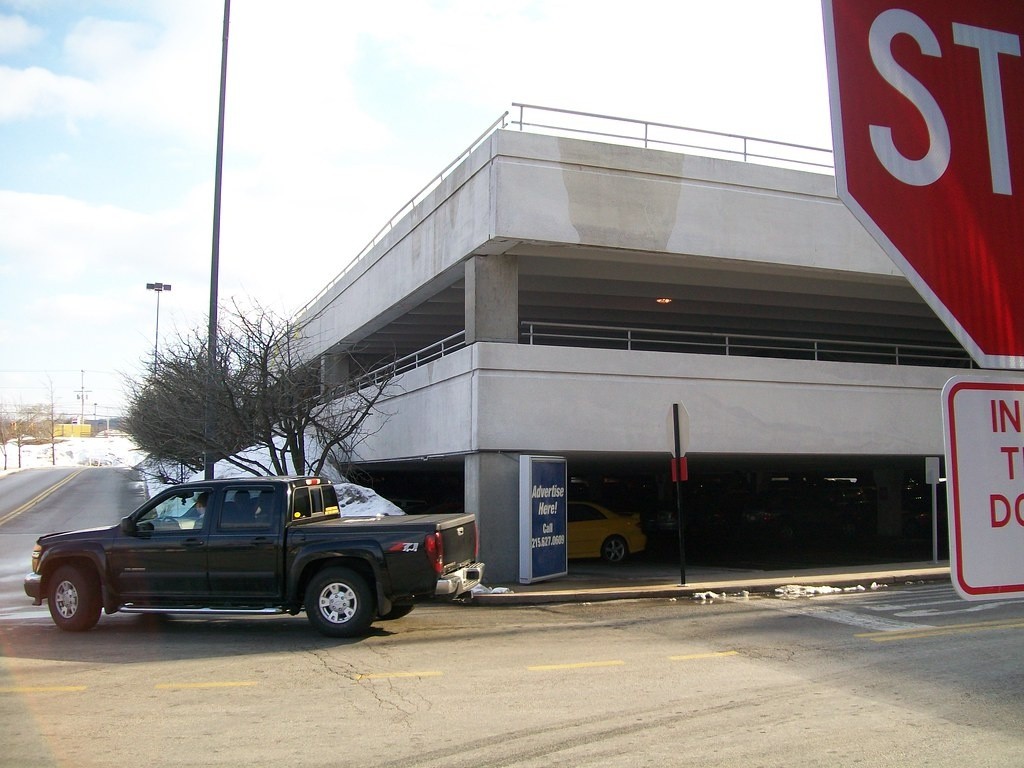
[189,491,277,529]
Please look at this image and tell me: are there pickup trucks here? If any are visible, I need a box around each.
[23,476,485,637]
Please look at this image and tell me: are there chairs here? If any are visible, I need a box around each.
[227,490,250,523]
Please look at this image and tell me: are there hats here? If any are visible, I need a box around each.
[197,493,207,507]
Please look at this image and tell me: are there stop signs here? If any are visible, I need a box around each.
[829,0,1024,371]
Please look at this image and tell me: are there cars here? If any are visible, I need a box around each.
[641,479,931,539]
[567,500,648,562]
[391,495,464,514]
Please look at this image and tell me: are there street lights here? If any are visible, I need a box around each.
[94,403,97,420]
[147,283,171,378]
[81,370,84,424]
